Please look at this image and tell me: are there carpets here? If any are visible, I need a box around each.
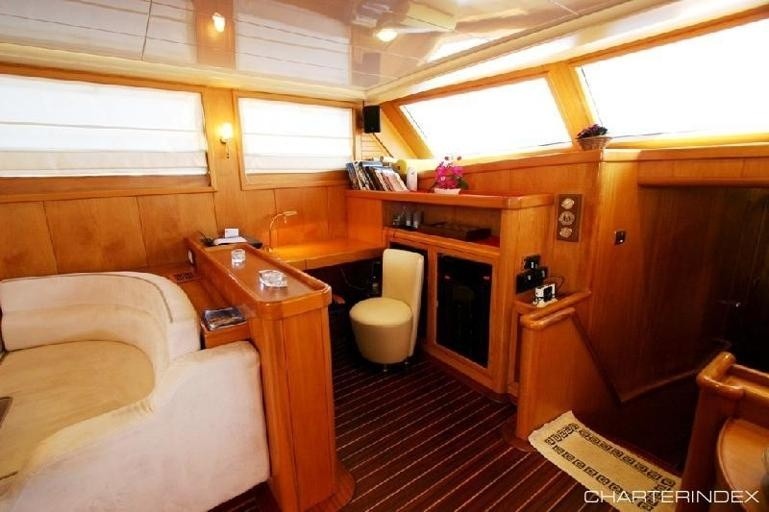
[528,410,682,512]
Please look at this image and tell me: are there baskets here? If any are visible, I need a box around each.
[575,136,614,151]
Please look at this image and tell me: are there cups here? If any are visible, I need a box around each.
[231,249,245,265]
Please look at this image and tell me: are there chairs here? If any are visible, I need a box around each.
[349,247,425,372]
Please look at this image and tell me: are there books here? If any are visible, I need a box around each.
[346,160,409,192]
[170,269,203,283]
[204,307,247,331]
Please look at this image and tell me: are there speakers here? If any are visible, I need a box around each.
[363,105,381,133]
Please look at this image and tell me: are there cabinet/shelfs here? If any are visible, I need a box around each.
[380,192,553,403]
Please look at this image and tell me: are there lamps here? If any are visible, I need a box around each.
[263,211,297,258]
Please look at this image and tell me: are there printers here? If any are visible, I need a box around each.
[211,235,262,249]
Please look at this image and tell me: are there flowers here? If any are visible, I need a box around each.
[429,156,468,190]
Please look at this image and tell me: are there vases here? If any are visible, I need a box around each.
[433,187,461,195]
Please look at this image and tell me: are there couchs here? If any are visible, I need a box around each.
[0,270,274,512]
